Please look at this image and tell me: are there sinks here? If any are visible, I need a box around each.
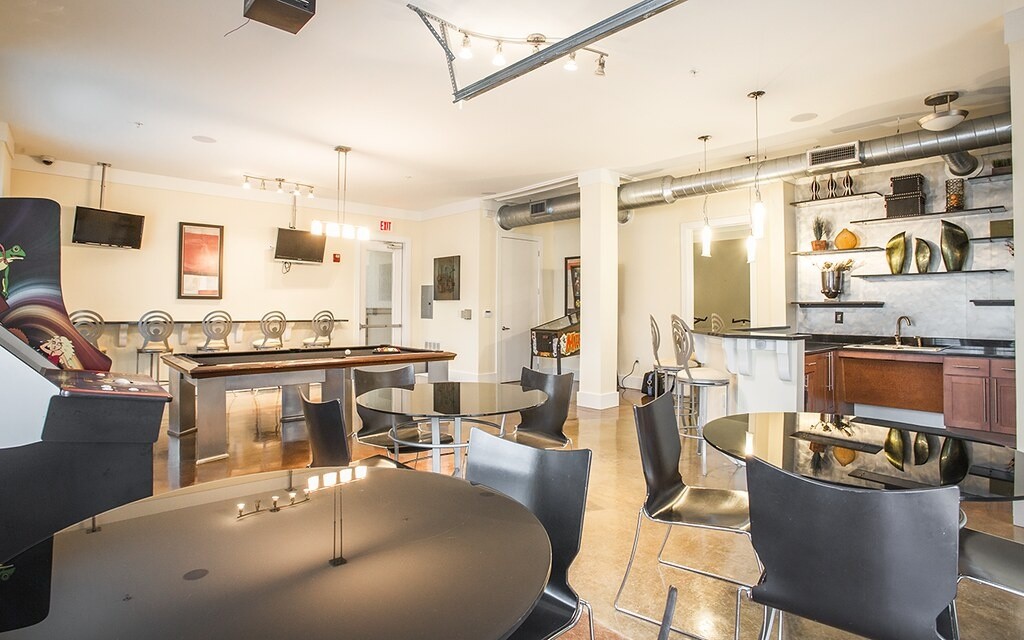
[843,344,948,352]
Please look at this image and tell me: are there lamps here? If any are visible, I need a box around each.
[745,153,758,265]
[233,466,366,566]
[698,133,715,257]
[749,91,767,238]
[404,3,612,76]
[243,171,315,200]
[916,90,968,132]
[305,144,376,243]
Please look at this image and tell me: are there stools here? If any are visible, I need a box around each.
[194,309,233,352]
[303,311,336,347]
[135,311,175,388]
[68,310,106,353]
[252,311,287,351]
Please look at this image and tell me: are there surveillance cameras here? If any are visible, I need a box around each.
[40,155,55,166]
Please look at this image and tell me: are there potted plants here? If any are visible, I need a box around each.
[810,216,829,251]
[991,158,1012,174]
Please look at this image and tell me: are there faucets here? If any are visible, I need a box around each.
[894,316,911,346]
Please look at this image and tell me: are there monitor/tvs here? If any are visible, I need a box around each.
[71,205,145,251]
[273,227,327,266]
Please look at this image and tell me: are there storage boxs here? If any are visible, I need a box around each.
[884,191,926,218]
[891,174,925,193]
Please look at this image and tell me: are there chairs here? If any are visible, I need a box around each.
[648,315,703,452]
[670,313,730,477]
[495,366,574,450]
[353,364,456,472]
[297,386,414,470]
[732,452,960,640]
[614,392,784,640]
[467,425,596,639]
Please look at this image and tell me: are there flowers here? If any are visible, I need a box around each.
[814,259,853,271]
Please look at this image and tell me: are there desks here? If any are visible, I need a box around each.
[70,317,349,348]
[157,343,456,462]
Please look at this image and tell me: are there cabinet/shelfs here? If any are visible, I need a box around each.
[793,174,1016,309]
[803,347,835,416]
[942,357,1016,436]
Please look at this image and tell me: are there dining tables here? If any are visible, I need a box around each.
[353,380,550,478]
[701,410,1024,500]
[1,459,556,640]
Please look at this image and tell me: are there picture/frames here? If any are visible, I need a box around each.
[178,222,224,301]
